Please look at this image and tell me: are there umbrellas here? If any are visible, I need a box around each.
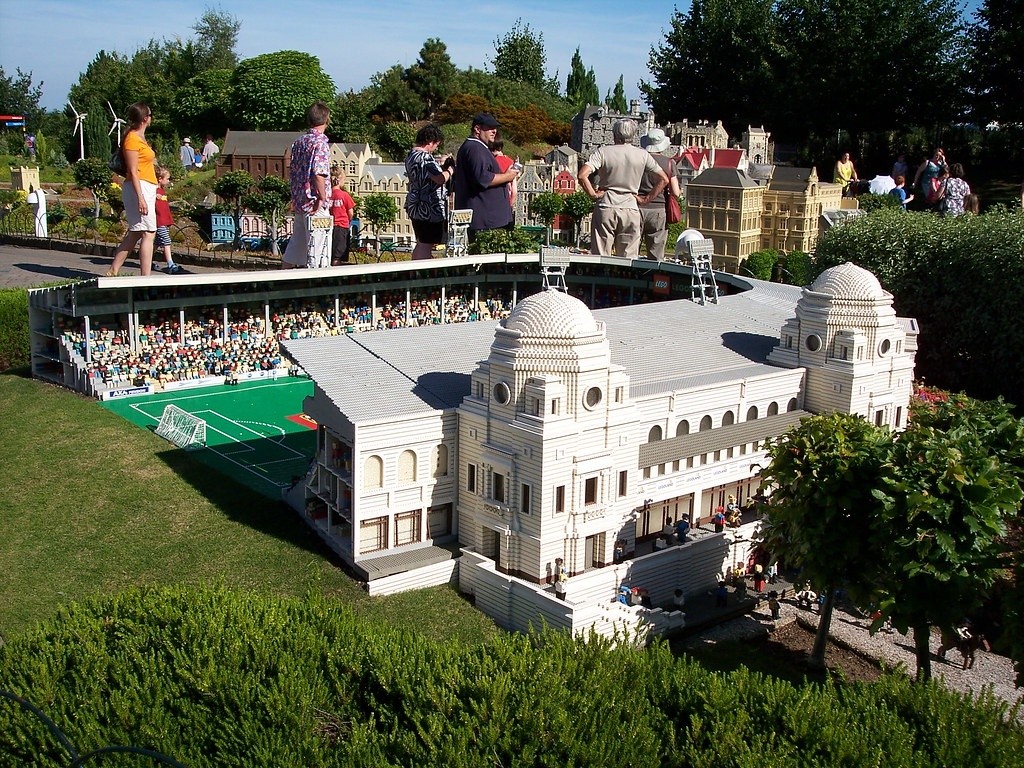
[868,175,896,194]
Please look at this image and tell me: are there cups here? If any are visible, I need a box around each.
[512,163,523,171]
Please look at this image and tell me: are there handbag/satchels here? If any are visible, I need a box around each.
[443,156,456,196]
[937,197,945,211]
[109,147,127,178]
[666,183,680,223]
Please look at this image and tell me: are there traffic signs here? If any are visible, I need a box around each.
[1,116,24,126]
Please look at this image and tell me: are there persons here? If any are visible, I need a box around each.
[554,494,973,651]
[107,100,158,278]
[403,124,455,260]
[912,147,946,208]
[281,103,332,269]
[833,150,857,192]
[892,153,908,184]
[926,164,970,219]
[329,167,356,267]
[57,266,652,392]
[889,176,914,211]
[24,131,38,162]
[639,128,680,261]
[153,165,182,275]
[456,113,519,238]
[578,118,669,259]
[180,138,220,177]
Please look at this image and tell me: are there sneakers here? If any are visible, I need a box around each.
[167,263,185,274]
[152,263,163,272]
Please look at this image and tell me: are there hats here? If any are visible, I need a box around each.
[472,113,503,127]
[677,229,704,254]
[641,128,670,152]
[182,138,192,144]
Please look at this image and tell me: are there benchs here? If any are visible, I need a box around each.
[58,291,510,400]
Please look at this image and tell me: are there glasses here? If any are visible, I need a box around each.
[433,141,440,147]
[146,114,152,117]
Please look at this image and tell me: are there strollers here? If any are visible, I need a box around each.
[845,179,870,197]
